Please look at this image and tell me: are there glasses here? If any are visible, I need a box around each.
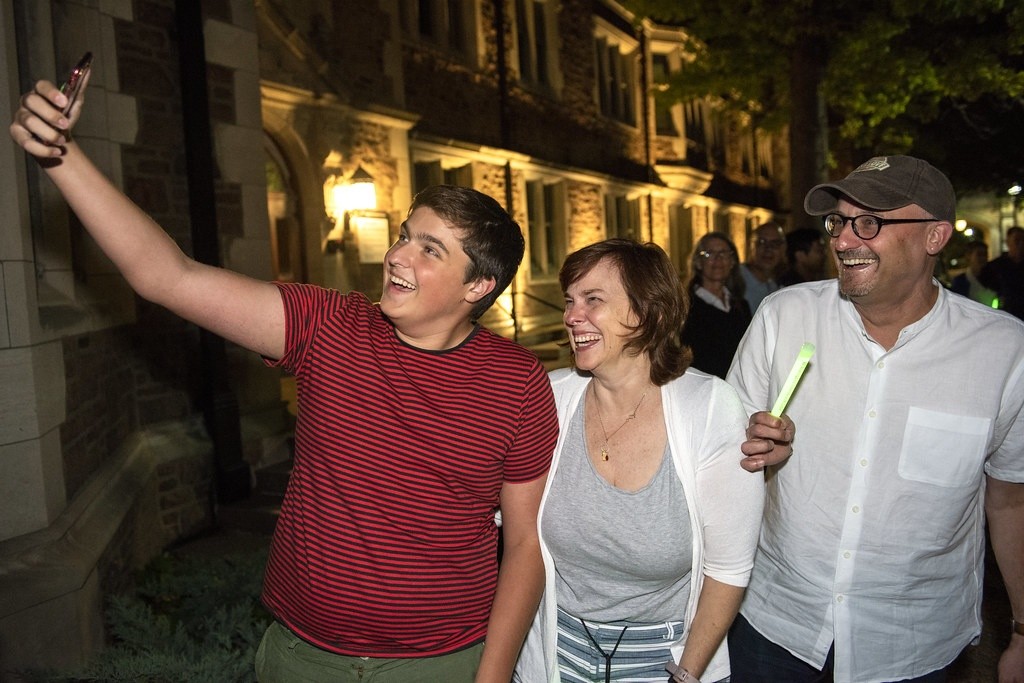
[701,251,731,261]
[758,239,784,248]
[822,213,939,240]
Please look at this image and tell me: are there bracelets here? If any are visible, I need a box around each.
[664,662,702,683]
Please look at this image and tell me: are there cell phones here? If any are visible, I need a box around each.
[35,51,94,147]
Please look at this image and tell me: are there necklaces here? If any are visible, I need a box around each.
[591,375,652,462]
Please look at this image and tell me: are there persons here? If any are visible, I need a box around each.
[684,220,1024,375]
[11,55,558,683]
[722,154,1024,683]
[491,234,763,683]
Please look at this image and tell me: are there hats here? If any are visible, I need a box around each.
[804,154,956,228]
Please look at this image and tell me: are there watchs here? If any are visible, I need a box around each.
[1009,618,1024,636]
